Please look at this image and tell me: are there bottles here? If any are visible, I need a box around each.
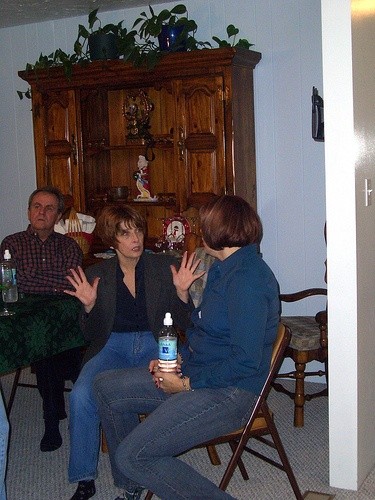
[1,250,18,303]
[158,312,177,374]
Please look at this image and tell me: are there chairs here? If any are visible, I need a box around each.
[8,322,303,500]
[271,221,328,427]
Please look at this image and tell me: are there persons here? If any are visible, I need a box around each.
[91,196,281,500]
[135,155,149,197]
[0,187,86,500]
[63,203,206,500]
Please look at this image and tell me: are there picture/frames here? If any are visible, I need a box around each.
[161,217,191,249]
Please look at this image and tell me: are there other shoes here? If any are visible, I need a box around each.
[70,480,95,500]
[115,486,148,500]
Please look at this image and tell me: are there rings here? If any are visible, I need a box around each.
[159,378,164,381]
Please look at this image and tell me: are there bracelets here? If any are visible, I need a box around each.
[182,375,192,392]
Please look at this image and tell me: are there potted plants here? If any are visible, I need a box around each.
[18,4,255,117]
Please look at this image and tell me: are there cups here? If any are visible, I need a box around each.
[108,186,131,200]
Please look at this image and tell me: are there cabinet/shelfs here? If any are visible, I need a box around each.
[18,48,258,271]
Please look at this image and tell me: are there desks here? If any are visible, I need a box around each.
[0,292,91,378]
